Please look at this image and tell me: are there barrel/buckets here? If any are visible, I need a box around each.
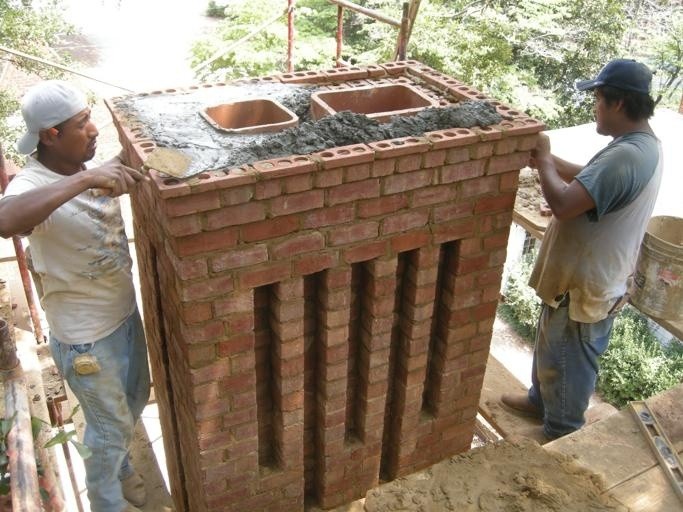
[631,216,683,320]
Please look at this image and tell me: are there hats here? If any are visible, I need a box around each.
[13,79,88,154]
[577,59,652,93]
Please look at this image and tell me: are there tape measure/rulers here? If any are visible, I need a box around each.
[71,354,100,377]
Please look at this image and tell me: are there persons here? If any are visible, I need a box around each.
[0,79,150,512]
[497,58,663,446]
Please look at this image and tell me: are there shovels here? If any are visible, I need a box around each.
[88,146,220,200]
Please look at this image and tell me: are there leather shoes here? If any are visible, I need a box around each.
[514,425,551,445]
[120,470,147,512]
[498,391,545,418]
